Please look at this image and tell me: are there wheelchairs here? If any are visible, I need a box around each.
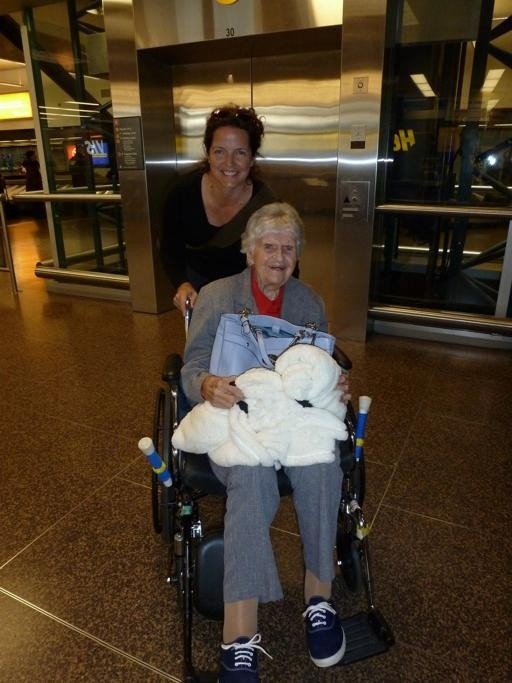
[137,292,396,682]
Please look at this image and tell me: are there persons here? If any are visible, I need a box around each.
[181,198,354,681]
[159,103,300,319]
[71,144,88,186]
[24,147,46,221]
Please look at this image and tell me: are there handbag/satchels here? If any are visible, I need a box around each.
[210,314,336,383]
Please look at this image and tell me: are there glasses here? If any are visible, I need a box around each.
[211,107,253,123]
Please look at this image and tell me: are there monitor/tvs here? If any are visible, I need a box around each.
[63,139,112,168]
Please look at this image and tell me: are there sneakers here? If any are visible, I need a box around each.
[221,635,258,683]
[300,595,345,669]
